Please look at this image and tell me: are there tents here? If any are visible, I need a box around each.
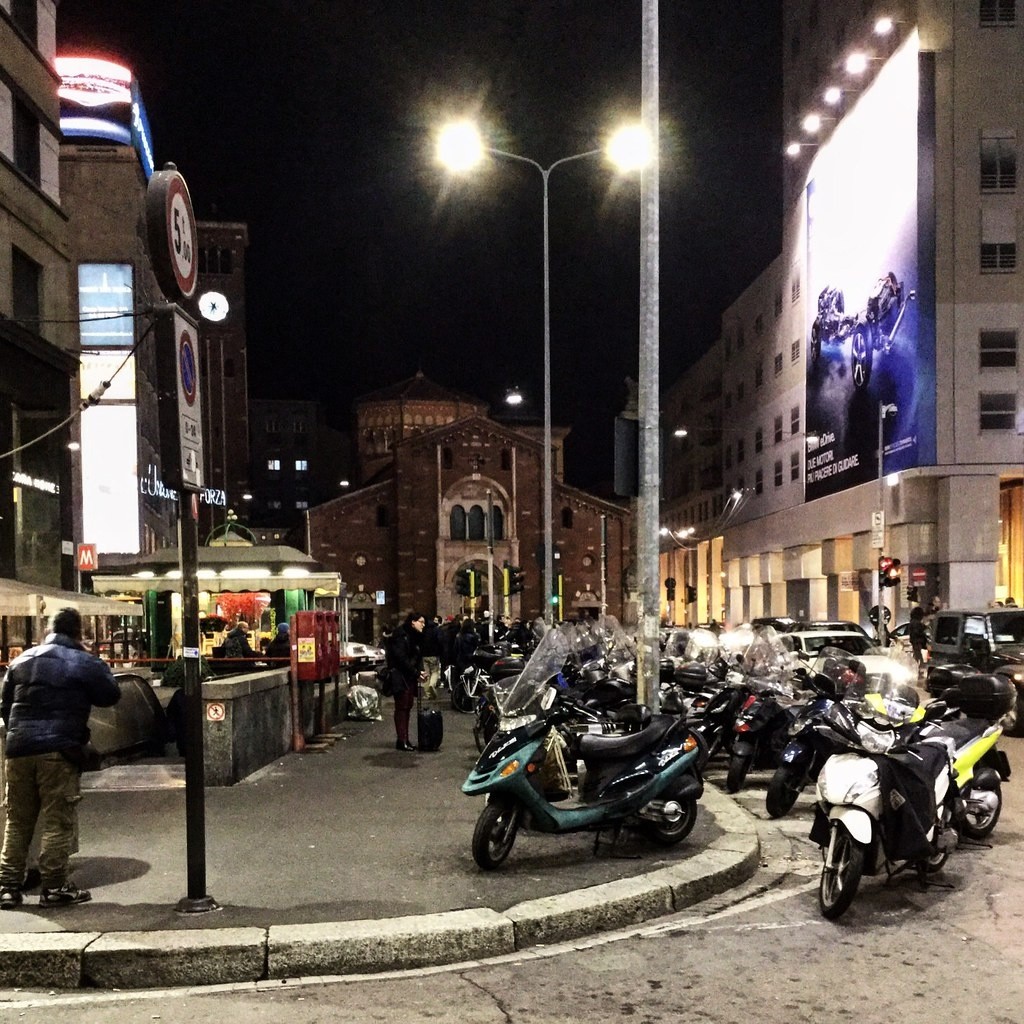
[0,574,147,666]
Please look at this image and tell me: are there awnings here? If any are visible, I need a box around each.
[93,564,342,596]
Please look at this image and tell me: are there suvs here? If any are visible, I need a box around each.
[926,607,1024,739]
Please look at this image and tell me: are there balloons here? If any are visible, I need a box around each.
[880,555,901,592]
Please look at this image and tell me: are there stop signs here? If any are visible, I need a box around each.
[912,568,927,581]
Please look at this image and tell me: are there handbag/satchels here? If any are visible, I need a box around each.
[380,669,396,696]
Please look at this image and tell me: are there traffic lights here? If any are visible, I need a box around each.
[688,585,698,603]
[907,585,918,602]
[878,557,903,587]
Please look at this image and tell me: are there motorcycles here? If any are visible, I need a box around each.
[447,616,1019,920]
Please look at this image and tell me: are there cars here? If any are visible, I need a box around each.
[889,622,930,667]
[775,631,913,690]
[779,622,882,652]
[751,616,798,634]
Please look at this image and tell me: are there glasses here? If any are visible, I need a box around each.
[416,620,425,625]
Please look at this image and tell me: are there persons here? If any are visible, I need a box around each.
[901,608,930,663]
[385,612,430,757]
[994,597,1018,608]
[266,622,292,658]
[0,608,122,910]
[432,608,536,713]
[218,618,264,665]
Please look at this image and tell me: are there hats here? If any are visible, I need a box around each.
[277,624,289,634]
[445,615,454,621]
[572,592,603,608]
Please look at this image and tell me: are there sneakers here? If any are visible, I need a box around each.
[39,880,91,906]
[0,882,23,908]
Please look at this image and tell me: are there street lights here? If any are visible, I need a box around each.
[436,124,646,623]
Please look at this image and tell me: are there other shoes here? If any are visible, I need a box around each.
[423,694,433,699]
[395,740,417,751]
[429,685,437,699]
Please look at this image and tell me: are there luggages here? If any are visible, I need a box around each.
[416,678,444,750]
